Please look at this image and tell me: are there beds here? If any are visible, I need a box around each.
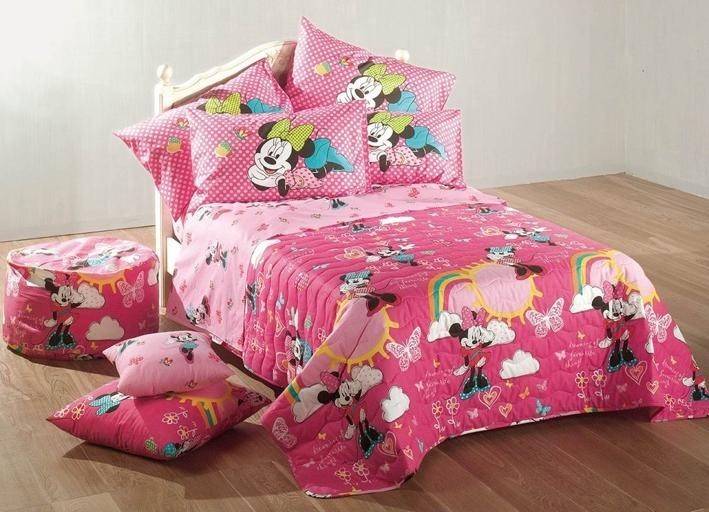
[150,43,651,482]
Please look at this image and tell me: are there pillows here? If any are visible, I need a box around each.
[44,330,273,463]
[282,17,456,112]
[113,58,294,221]
[184,99,371,214]
[369,109,465,191]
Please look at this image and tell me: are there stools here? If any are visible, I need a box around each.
[1,236,161,364]
